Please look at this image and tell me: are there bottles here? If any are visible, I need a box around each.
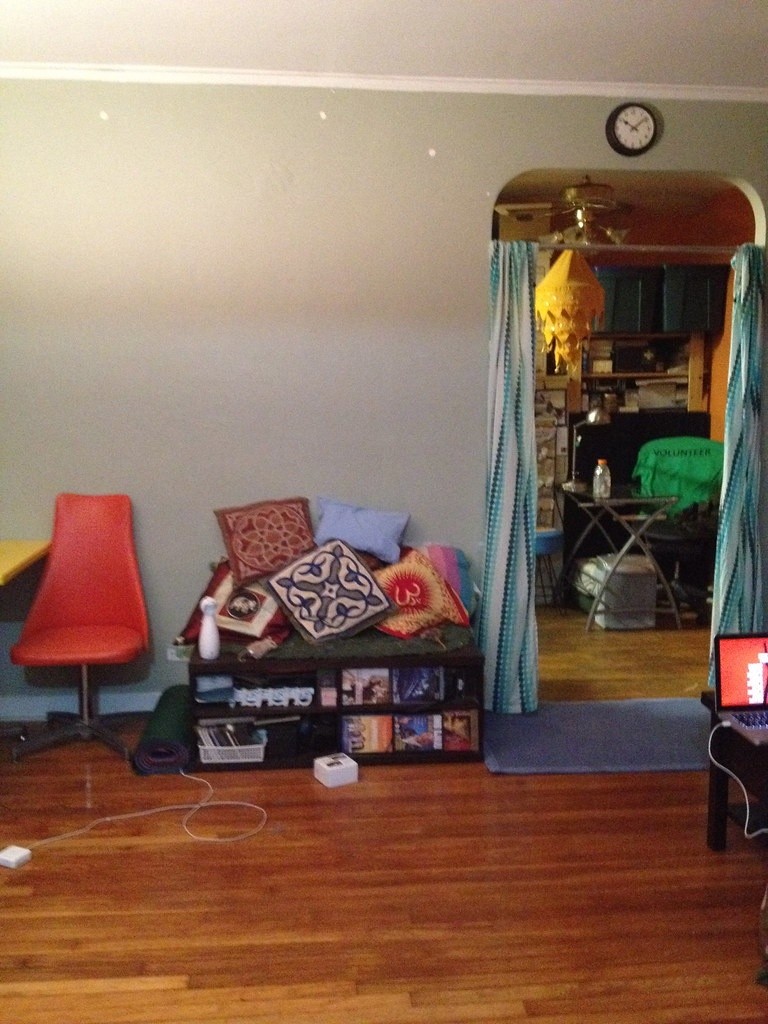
[593,459,611,498]
[197,596,220,659]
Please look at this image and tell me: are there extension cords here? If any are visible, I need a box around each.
[0,845,32,870]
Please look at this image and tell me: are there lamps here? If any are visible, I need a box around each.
[537,173,633,253]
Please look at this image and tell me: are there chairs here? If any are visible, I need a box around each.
[11,483,152,763]
[632,434,728,620]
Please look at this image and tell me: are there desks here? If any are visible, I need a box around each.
[554,480,686,634]
[0,538,51,586]
[698,689,768,854]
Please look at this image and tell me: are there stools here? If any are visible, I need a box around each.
[536,528,568,616]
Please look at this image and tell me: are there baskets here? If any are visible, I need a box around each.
[198,731,266,762]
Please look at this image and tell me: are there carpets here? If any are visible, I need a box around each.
[482,691,712,776]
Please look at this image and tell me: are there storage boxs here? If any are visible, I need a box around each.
[637,381,679,410]
[593,265,655,333]
[199,718,268,767]
[592,554,656,633]
[497,202,554,244]
[591,356,616,374]
[656,259,732,338]
[310,750,359,787]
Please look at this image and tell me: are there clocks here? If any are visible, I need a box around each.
[606,102,657,157]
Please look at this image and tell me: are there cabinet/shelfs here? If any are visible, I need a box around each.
[193,619,489,774]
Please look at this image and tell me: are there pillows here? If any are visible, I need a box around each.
[374,552,460,642]
[176,557,292,650]
[212,496,323,589]
[314,495,415,566]
[407,541,477,614]
[262,535,397,645]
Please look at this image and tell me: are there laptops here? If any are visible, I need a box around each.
[713,631,767,746]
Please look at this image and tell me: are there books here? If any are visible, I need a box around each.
[197,726,245,760]
[341,666,480,752]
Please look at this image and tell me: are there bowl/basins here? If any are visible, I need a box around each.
[536,532,561,555]
